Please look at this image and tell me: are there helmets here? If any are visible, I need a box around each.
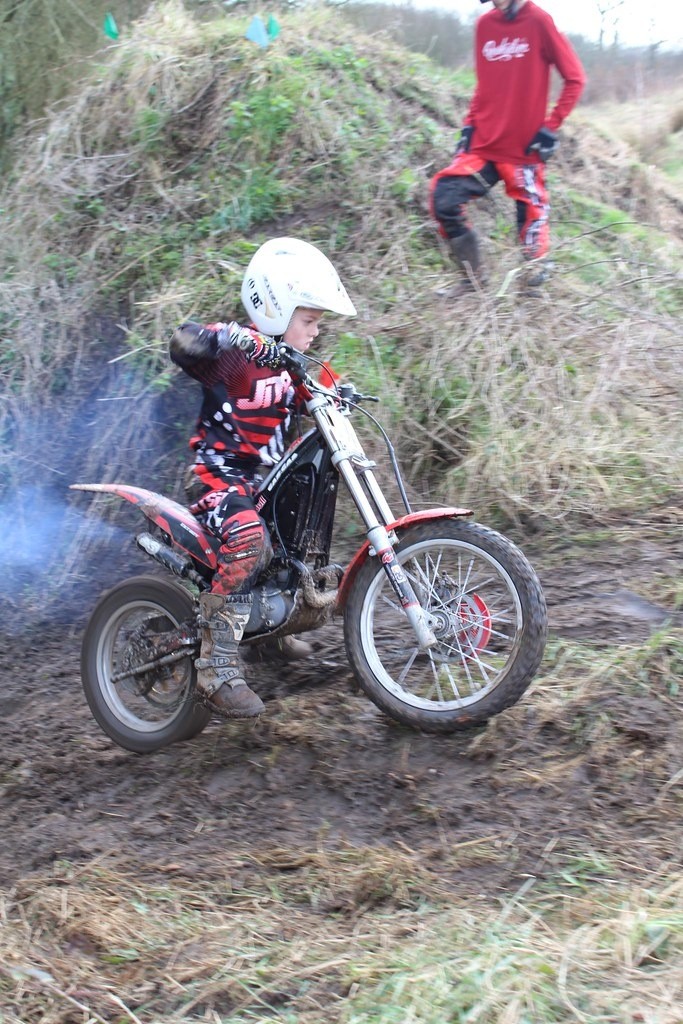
[243,237,357,335]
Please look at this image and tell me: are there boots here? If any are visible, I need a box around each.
[519,254,545,305]
[445,233,489,300]
[254,635,312,658]
[196,591,268,716]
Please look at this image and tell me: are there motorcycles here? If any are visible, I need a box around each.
[65,329,548,758]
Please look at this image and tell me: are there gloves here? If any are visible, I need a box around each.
[526,124,557,158]
[219,324,279,367]
[454,127,475,155]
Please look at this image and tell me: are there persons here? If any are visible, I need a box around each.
[165,233,359,721]
[421,1,586,295]
